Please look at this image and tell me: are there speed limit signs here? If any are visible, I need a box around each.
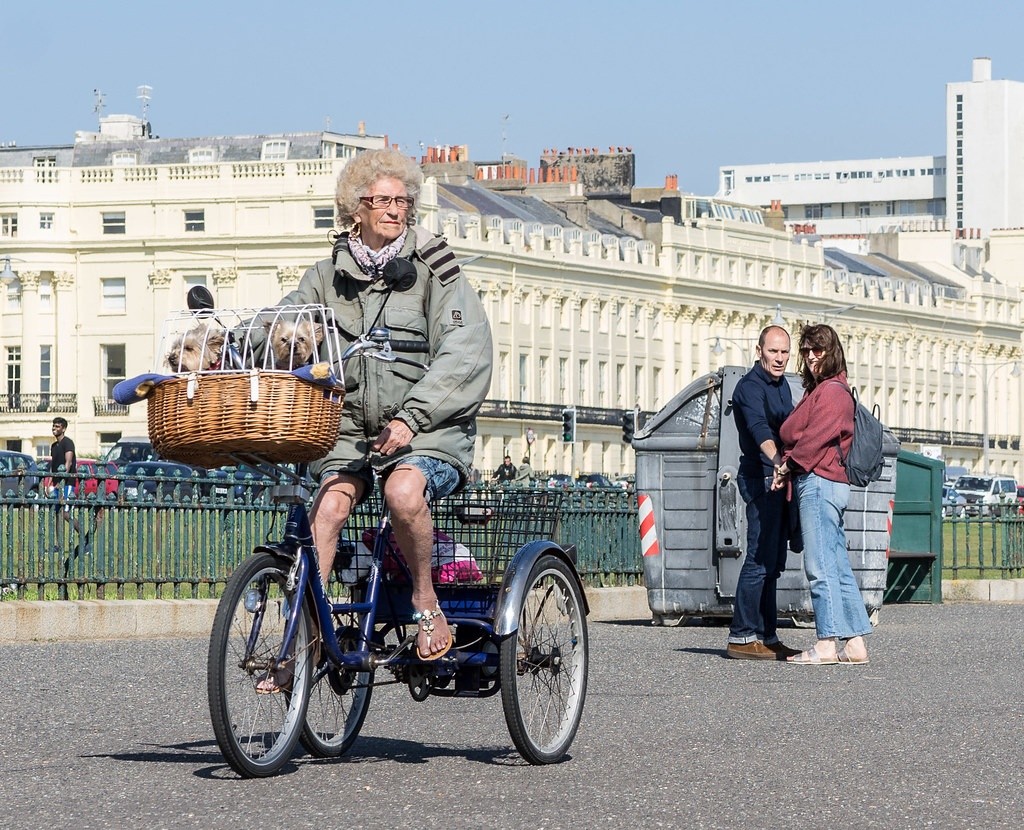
[526,430,534,445]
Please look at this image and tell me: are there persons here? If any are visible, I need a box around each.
[492,455,518,484]
[728,326,803,660]
[230,147,493,694]
[516,457,537,489]
[124,447,134,462]
[50,418,78,551]
[770,324,872,664]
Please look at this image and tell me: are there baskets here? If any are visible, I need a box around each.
[147,309,344,468]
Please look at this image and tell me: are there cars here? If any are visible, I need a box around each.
[118,462,196,501]
[942,485,967,517]
[0,450,39,499]
[543,474,629,489]
[1014,485,1024,515]
[202,462,292,504]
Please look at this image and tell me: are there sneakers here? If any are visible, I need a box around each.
[765,641,803,661]
[726,640,785,661]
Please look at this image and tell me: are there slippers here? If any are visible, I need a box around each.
[786,647,839,665]
[254,659,295,694]
[413,596,452,661]
[835,648,871,664]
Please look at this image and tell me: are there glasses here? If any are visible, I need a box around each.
[359,194,415,209]
[800,346,826,358]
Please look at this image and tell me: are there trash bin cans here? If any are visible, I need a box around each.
[630,364,901,630]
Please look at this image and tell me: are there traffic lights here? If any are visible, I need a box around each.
[622,412,635,444]
[560,408,577,445]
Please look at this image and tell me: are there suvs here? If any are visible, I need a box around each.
[37,459,121,498]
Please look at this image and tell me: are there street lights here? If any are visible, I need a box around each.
[953,359,1021,477]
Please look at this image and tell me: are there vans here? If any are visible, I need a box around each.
[949,475,1017,517]
[103,436,158,462]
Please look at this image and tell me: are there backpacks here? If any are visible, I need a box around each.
[827,379,885,487]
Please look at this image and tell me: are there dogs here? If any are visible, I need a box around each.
[263,320,322,369]
[167,322,233,371]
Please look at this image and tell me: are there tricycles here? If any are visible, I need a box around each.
[145,302,592,780]
